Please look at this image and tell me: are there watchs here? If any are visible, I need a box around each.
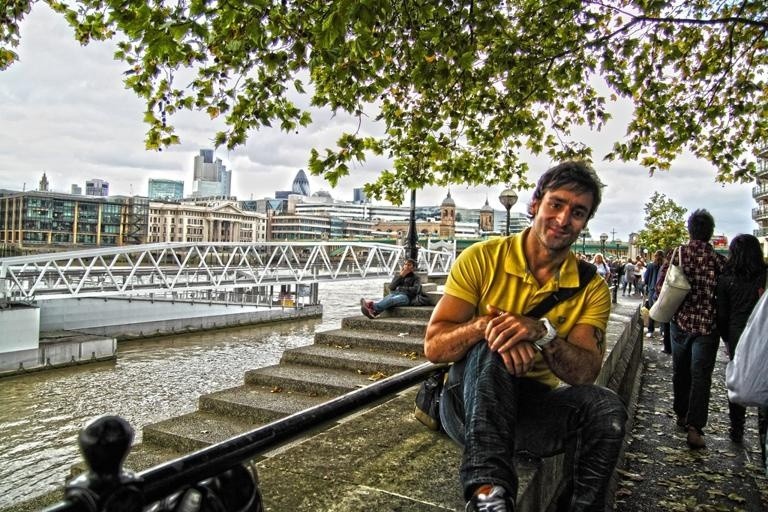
[533,317,558,351]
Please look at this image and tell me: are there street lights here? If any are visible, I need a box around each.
[615,238,622,259]
[500,190,517,237]
[600,233,608,259]
[580,231,588,256]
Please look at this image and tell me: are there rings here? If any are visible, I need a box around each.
[497,309,504,315]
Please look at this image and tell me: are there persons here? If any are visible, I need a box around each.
[361,260,420,319]
[652,208,729,448]
[424,160,628,512]
[574,250,672,353]
[715,234,768,467]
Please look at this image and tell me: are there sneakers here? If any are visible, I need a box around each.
[646,333,653,338]
[675,415,705,447]
[661,348,671,354]
[361,298,377,319]
[471,487,514,512]
[729,428,743,442]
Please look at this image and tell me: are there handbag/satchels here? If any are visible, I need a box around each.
[411,286,432,306]
[649,264,691,324]
[414,369,448,431]
[640,306,660,328]
